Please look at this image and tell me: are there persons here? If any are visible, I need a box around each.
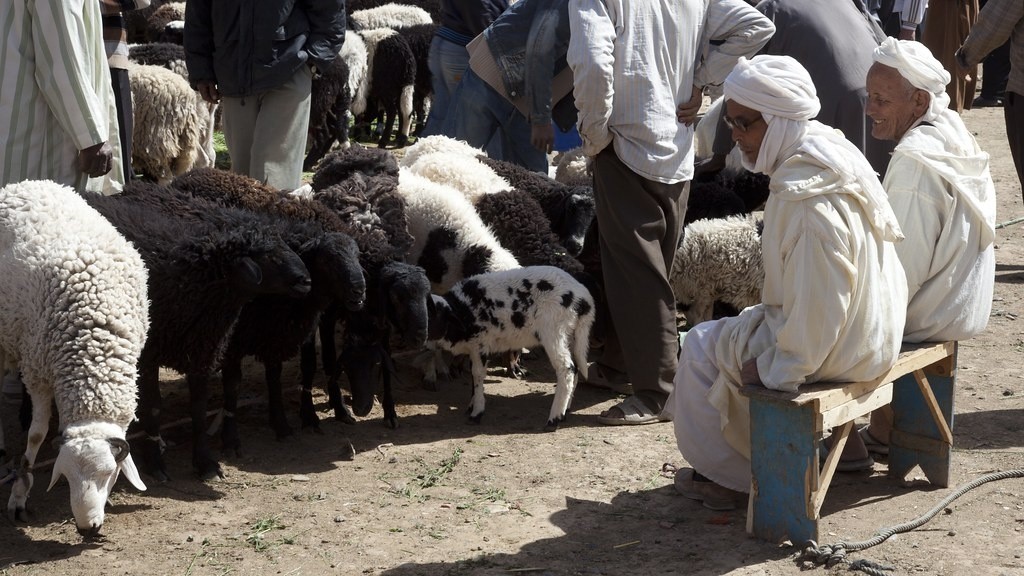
[823,37,997,474]
[99,0,1012,162]
[659,54,908,498]
[954,0,1024,200]
[565,0,778,426]
[0,0,128,197]
[183,0,347,197]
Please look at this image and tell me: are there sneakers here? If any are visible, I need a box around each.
[674,467,736,509]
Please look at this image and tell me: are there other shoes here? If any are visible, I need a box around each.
[972,96,998,107]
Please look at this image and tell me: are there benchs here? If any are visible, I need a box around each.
[739,342,959,544]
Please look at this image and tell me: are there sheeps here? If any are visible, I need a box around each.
[311,133,770,377]
[424,264,595,430]
[0,176,150,539]
[121,0,438,184]
[85,177,310,484]
[173,168,368,434]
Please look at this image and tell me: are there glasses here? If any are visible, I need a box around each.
[723,114,761,132]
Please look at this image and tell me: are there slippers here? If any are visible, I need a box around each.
[857,423,889,455]
[577,362,635,396]
[596,395,666,425]
[819,439,874,472]
